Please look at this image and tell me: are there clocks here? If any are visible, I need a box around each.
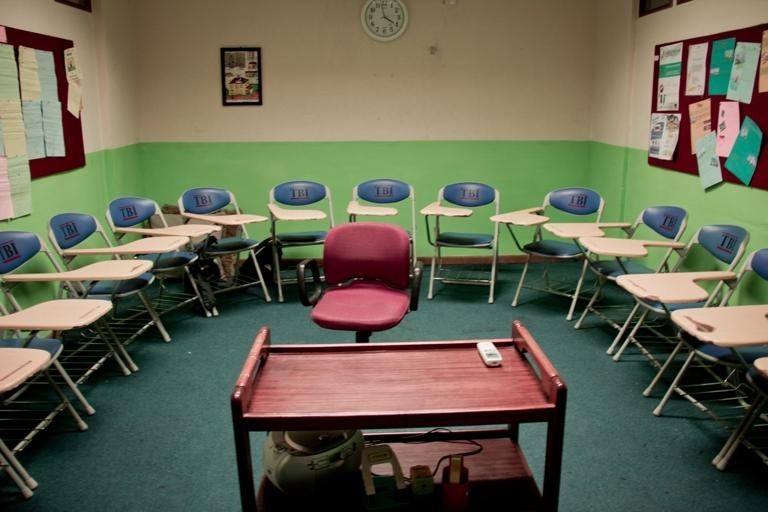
[360,1,409,42]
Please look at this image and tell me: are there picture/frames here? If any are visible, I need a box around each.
[220,46,262,105]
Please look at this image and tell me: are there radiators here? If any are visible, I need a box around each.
[477,341,502,367]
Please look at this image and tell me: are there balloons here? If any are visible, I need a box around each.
[360,1,409,42]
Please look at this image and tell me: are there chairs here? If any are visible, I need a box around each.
[105,196,222,317]
[266,180,334,303]
[491,185,605,321]
[346,178,417,268]
[296,222,425,343]
[579,224,750,397]
[420,181,501,304]
[48,212,191,372]
[1,231,153,414]
[653,248,768,469]
[542,205,689,356]
[178,186,269,316]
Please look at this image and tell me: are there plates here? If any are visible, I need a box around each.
[230,319,568,512]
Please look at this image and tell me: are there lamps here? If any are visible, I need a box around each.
[296,222,425,343]
[266,180,334,303]
[346,178,417,268]
[420,181,501,304]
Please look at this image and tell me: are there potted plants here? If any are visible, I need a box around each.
[220,46,262,105]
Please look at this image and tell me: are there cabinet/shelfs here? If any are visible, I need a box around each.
[230,319,568,512]
